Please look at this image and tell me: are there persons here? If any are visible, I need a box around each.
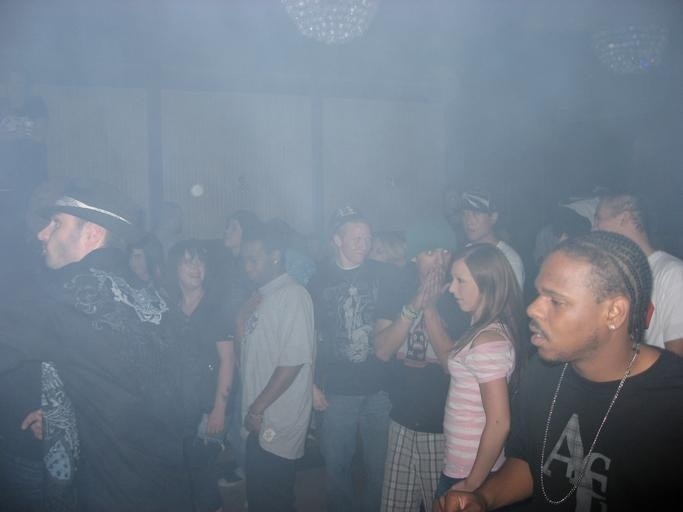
[230,217,318,512]
[166,240,235,512]
[128,231,161,284]
[590,190,683,358]
[217,209,257,323]
[371,215,469,512]
[0,179,219,512]
[305,205,393,511]
[458,186,526,295]
[431,229,683,512]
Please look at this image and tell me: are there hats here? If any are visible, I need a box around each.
[40,181,141,239]
[400,215,458,259]
[461,185,499,215]
[325,203,365,228]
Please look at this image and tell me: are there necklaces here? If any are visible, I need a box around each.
[540,339,641,505]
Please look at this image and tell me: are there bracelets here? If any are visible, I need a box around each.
[400,302,418,323]
[245,406,262,420]
